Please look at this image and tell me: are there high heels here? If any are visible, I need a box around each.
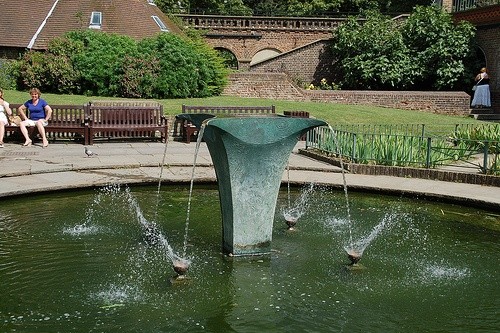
[43,141,48,147]
[23,140,32,146]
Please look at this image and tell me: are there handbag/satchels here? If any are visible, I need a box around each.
[8,115,22,126]
[472,86,476,91]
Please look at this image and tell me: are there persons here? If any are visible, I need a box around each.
[471,67,491,109]
[19,88,52,147]
[0,90,12,148]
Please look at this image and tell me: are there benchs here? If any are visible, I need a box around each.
[2,103,169,145]
[181,104,276,144]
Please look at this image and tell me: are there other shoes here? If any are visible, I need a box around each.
[0,142,5,148]
[475,106,482,108]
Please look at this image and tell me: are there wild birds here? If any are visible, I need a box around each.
[84,147,98,157]
